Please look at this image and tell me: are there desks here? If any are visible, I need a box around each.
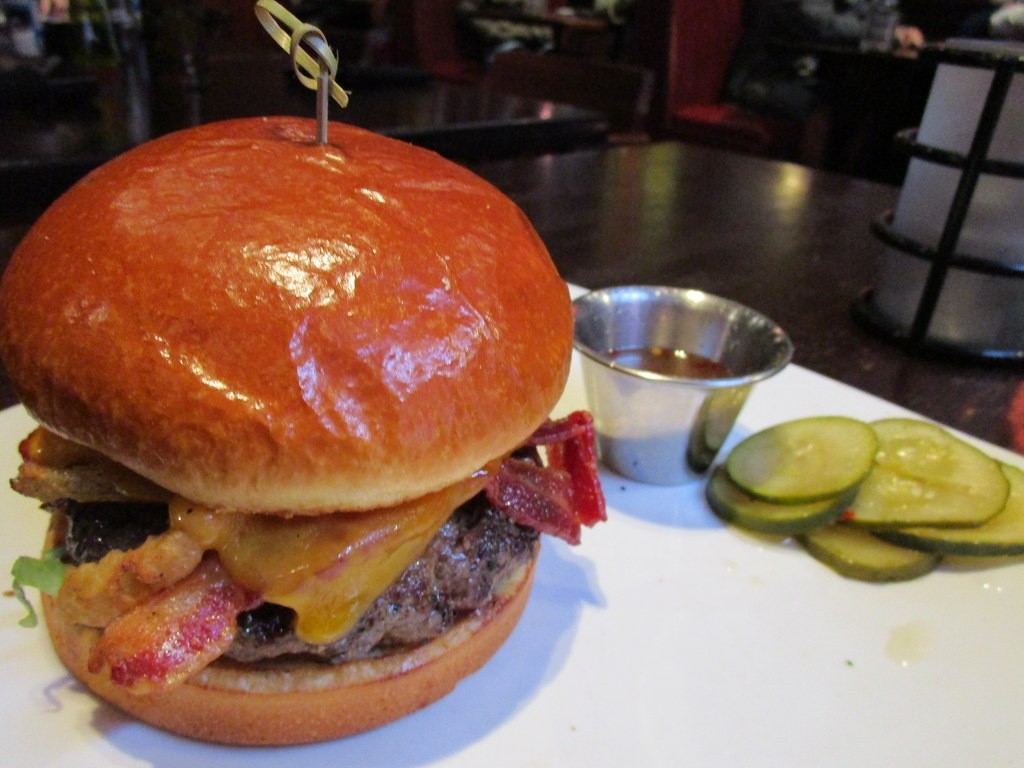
[0,281,1024,768]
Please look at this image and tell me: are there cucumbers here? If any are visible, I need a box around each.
[706,416,1024,581]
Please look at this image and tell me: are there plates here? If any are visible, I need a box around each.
[0,282,1024,768]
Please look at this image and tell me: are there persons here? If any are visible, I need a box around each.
[720,0,1024,184]
[455,0,671,69]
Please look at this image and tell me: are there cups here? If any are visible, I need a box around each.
[571,286,793,487]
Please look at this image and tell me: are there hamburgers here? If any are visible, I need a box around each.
[0,114,603,746]
